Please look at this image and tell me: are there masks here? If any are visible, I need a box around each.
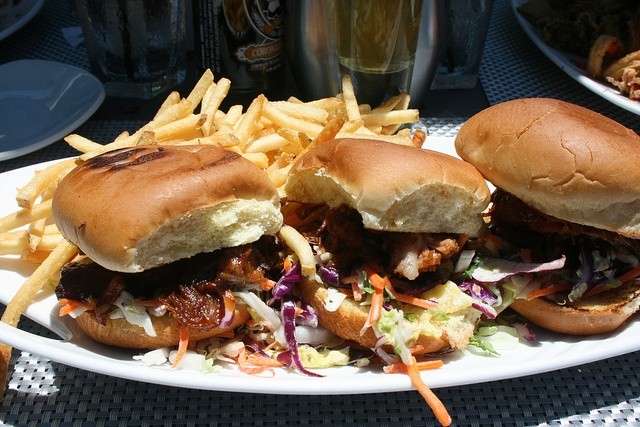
[52,63,422,175]
[0,161,74,365]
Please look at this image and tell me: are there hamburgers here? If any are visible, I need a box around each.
[50,144,284,349]
[284,138,491,356]
[454,97,636,336]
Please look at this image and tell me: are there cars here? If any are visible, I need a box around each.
[430,0,493,91]
[67,0,198,101]
[330,2,423,111]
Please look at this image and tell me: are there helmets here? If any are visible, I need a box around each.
[0,137,639,395]
[511,0,640,117]
[0,59,106,162]
